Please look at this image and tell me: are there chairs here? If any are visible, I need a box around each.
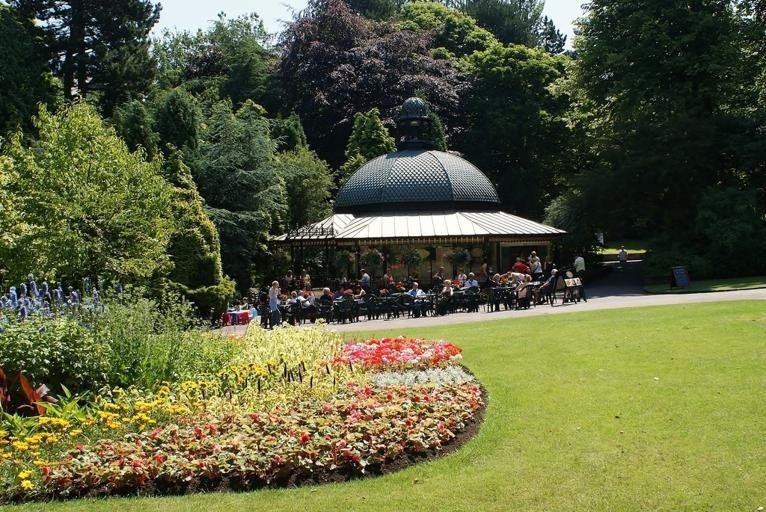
[254,271,561,324]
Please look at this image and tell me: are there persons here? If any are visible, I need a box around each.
[300,268,311,286]
[615,245,629,273]
[281,286,317,324]
[358,268,370,287]
[512,251,553,281]
[241,297,248,310]
[433,267,478,317]
[408,282,427,318]
[492,271,532,310]
[384,268,393,284]
[285,269,293,287]
[320,284,367,323]
[537,269,558,305]
[260,281,287,329]
[573,251,586,286]
